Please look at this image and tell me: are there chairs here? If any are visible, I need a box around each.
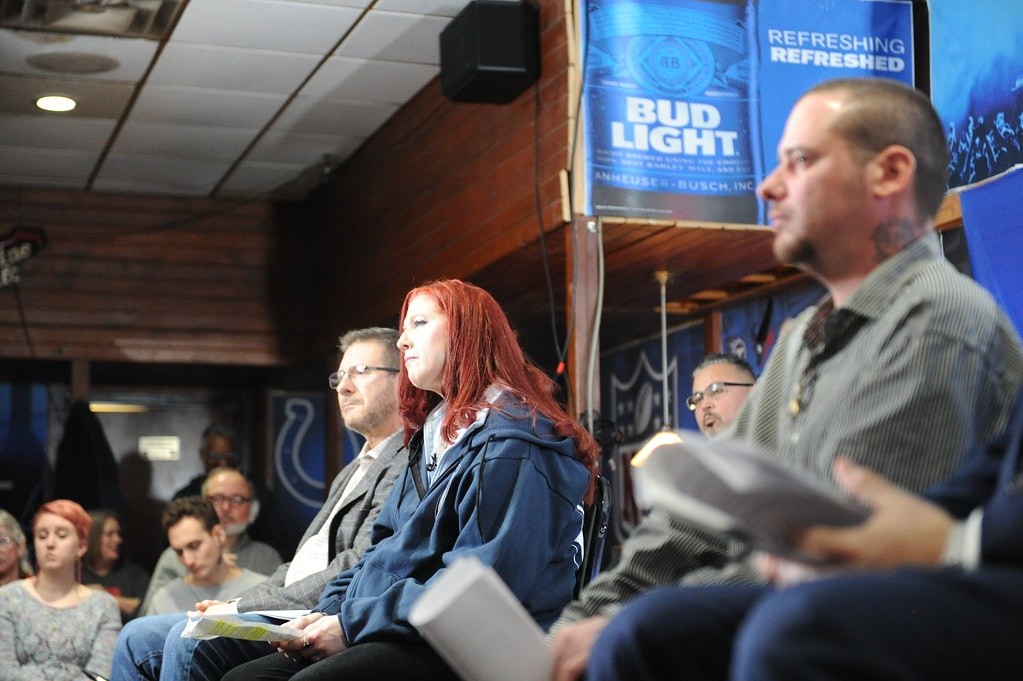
[573,475,614,601]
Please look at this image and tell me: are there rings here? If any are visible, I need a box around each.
[303,635,311,649]
[315,653,321,661]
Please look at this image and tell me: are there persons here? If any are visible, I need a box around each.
[3,500,122,681]
[221,277,601,681]
[112,326,427,681]
[171,421,265,541]
[538,77,1022,681]
[139,466,284,622]
[585,372,1023,681]
[147,497,273,618]
[687,353,757,439]
[0,510,33,586]
[81,508,150,620]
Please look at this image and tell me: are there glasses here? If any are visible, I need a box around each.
[686,382,753,411]
[328,364,400,389]
[0,535,16,546]
[208,494,250,504]
[206,450,239,466]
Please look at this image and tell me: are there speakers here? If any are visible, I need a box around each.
[439,0,542,105]
